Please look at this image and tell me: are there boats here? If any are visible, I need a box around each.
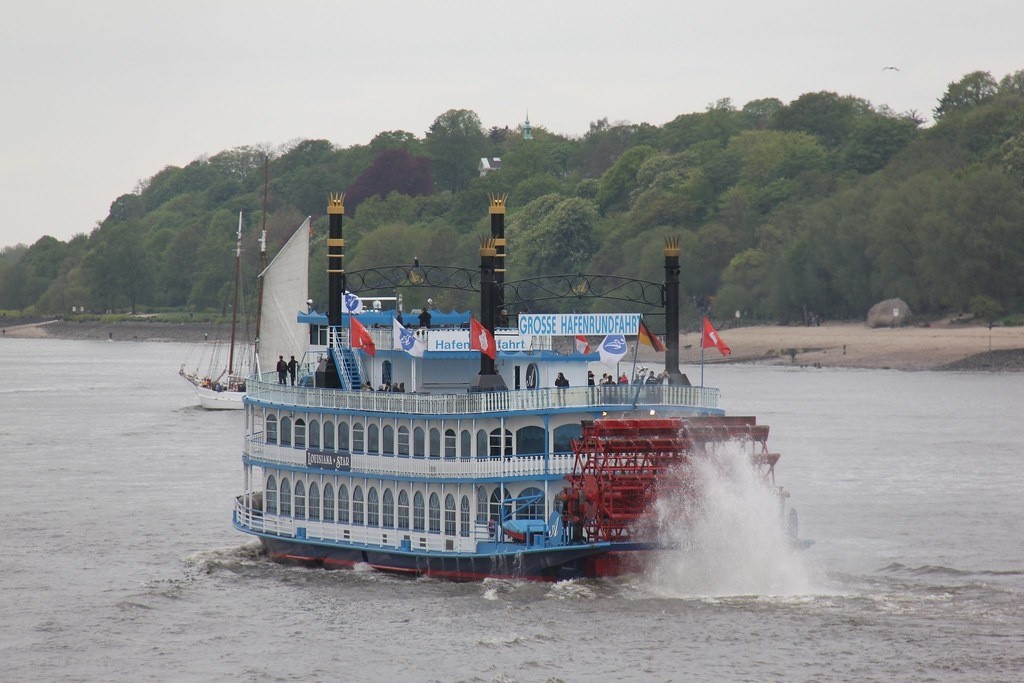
[230,190,815,583]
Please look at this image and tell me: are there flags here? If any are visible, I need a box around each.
[702,317,732,358]
[597,332,628,367]
[638,320,668,352]
[471,318,496,360]
[340,292,364,315]
[393,319,428,359]
[350,316,376,357]
[576,333,590,355]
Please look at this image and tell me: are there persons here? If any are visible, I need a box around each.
[555,373,569,386]
[496,309,510,328]
[361,381,405,393]
[276,355,288,385]
[417,307,431,328]
[200,378,222,393]
[287,356,298,386]
[586,370,663,386]
[396,311,404,326]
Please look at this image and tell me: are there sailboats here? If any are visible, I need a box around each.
[176,157,314,412]
